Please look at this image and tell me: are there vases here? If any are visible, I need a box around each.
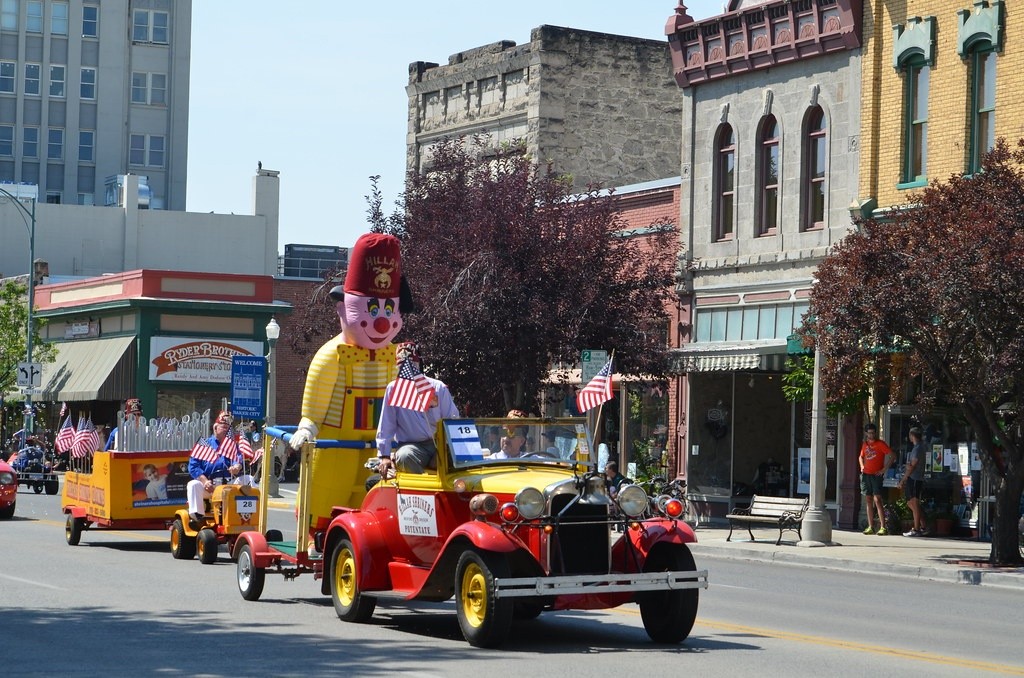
[875,515,953,535]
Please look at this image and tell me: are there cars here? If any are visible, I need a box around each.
[314,413,710,650]
[0,458,19,518]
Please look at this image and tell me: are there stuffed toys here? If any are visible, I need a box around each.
[288,233,413,556]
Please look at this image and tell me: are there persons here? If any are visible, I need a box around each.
[143,464,168,500]
[489,410,545,459]
[606,460,634,530]
[187,410,243,532]
[375,341,460,483]
[105,398,143,452]
[859,424,931,536]
[540,430,560,458]
[489,426,501,454]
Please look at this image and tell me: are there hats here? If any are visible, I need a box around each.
[501,409,530,430]
[910,427,922,434]
[541,429,556,442]
[396,342,419,363]
[215,410,232,425]
[125,398,141,414]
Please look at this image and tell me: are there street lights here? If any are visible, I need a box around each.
[264,314,281,495]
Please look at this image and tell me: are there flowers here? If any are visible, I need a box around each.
[873,497,954,528]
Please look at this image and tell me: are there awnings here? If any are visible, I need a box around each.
[673,353,814,376]
[3,336,137,402]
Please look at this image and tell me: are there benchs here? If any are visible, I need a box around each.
[726,493,809,546]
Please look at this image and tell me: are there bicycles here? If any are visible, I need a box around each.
[661,481,699,530]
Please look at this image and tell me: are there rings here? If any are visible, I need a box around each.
[379,469,382,472]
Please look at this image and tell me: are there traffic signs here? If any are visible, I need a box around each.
[17,363,30,386]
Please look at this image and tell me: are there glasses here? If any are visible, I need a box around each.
[498,427,522,438]
[134,413,140,417]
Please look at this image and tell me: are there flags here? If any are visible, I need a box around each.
[190,436,219,464]
[576,359,615,414]
[239,429,254,460]
[250,437,277,466]
[387,350,435,413]
[216,434,239,464]
[54,414,99,458]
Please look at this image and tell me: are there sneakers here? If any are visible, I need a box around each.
[863,527,876,535]
[902,527,922,537]
[919,527,930,536]
[877,527,888,536]
